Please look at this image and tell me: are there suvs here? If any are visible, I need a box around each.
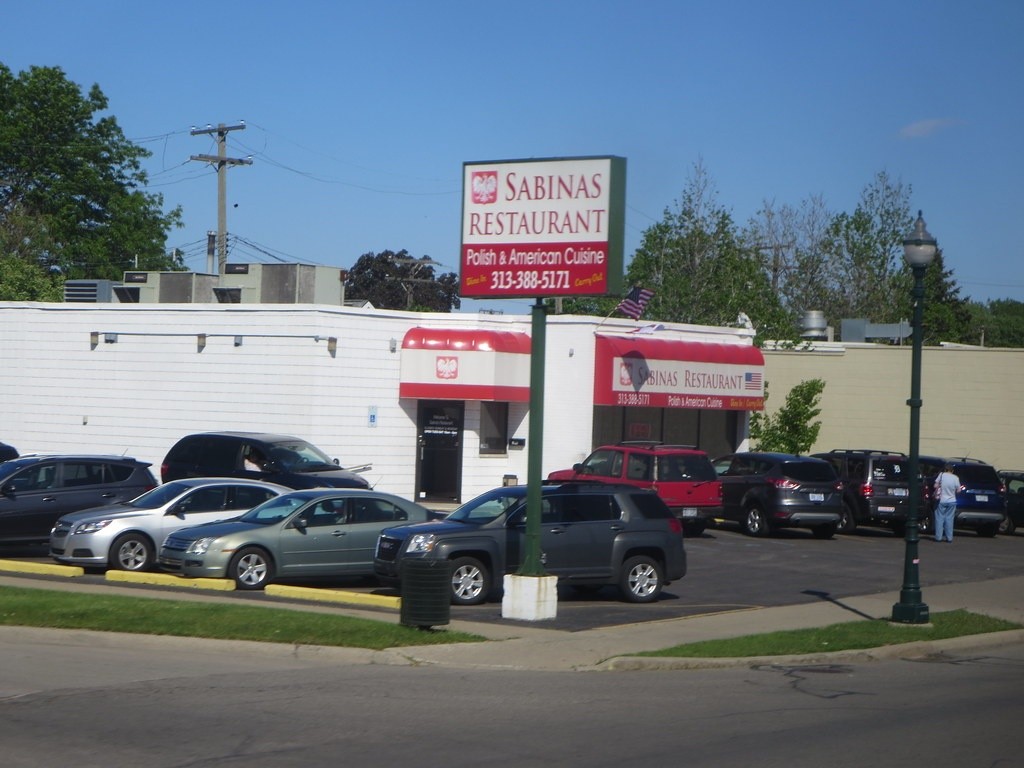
[0,456,160,546]
[903,454,1006,538]
[371,479,688,603]
[708,452,846,540]
[545,440,727,536]
[161,432,374,513]
[809,447,930,537]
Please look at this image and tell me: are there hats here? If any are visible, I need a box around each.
[945,463,953,470]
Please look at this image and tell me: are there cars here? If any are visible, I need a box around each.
[7,454,57,491]
[998,469,1024,534]
[155,489,451,590]
[0,443,19,464]
[48,477,325,573]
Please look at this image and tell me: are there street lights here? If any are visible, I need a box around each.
[891,210,939,625]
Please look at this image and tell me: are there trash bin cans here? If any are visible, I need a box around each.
[400,557,450,633]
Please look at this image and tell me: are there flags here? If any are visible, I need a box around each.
[615,289,656,320]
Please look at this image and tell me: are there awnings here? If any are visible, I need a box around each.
[595,334,764,412]
[400,328,532,402]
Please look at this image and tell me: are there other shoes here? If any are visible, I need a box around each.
[935,540,941,542]
[945,540,952,543]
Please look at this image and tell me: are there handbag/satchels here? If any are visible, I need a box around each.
[931,471,944,501]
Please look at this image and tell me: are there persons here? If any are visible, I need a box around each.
[933,465,961,543]
[244,448,271,472]
